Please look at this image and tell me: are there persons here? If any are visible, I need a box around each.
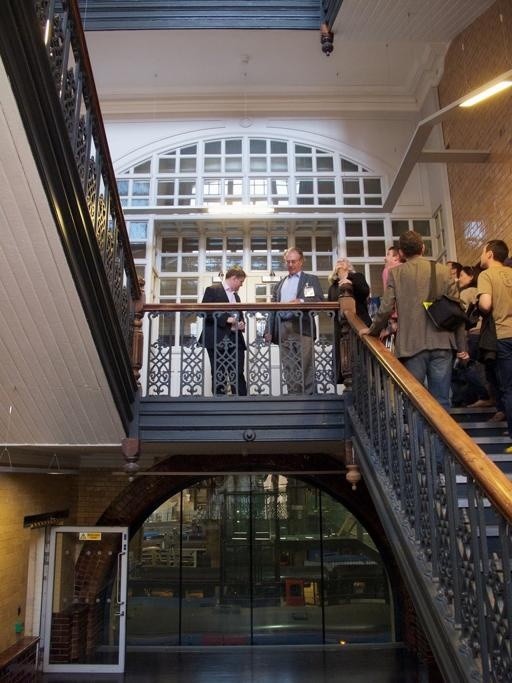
[264,246,324,394]
[325,258,371,383]
[446,259,461,298]
[476,239,512,453]
[452,266,494,407]
[377,245,401,344]
[359,228,470,494]
[195,270,250,394]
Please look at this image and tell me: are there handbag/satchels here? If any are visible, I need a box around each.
[421,292,470,333]
